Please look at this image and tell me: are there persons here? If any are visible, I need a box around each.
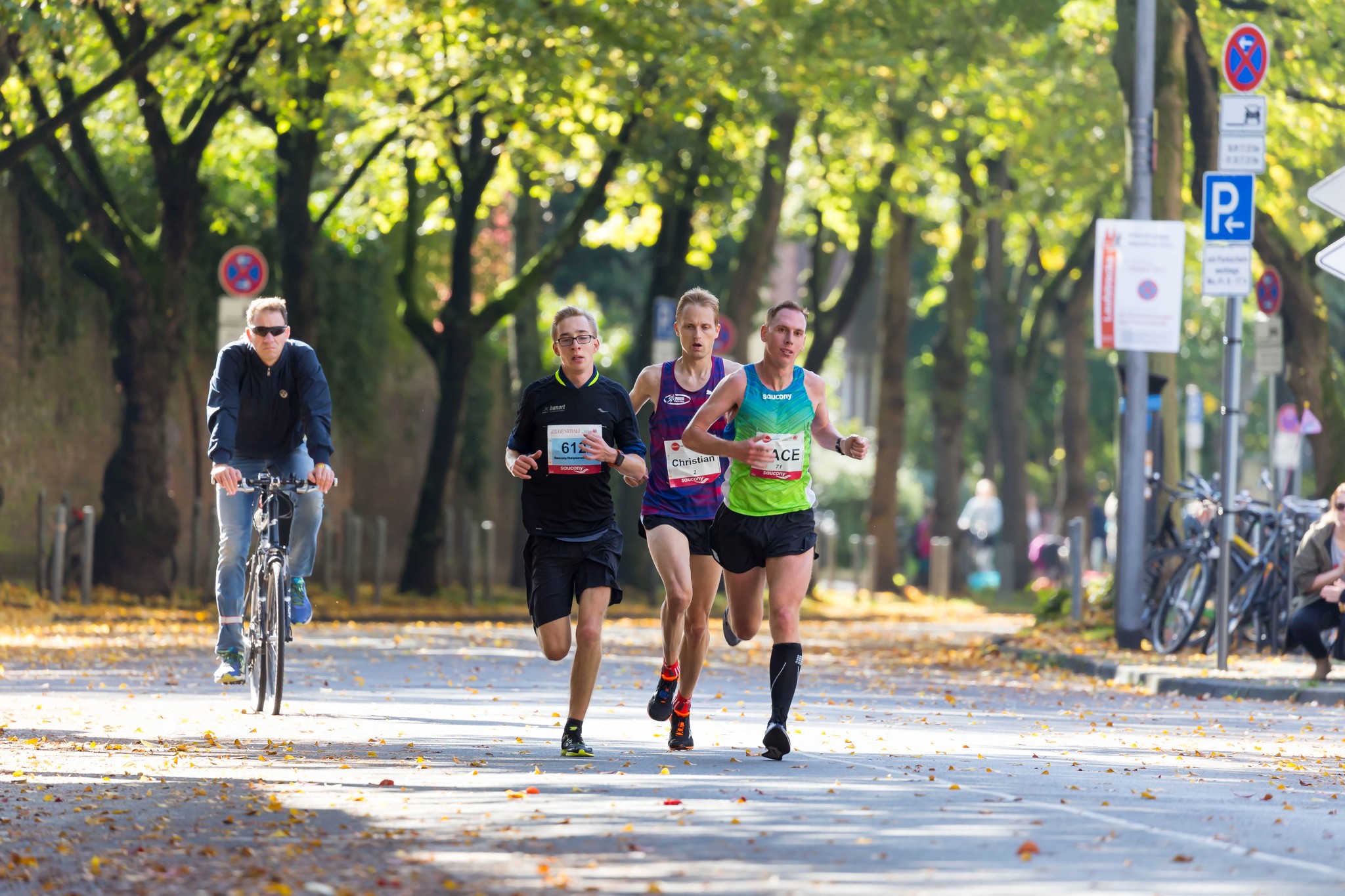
[681,300,870,761]
[504,307,646,757]
[206,296,336,684]
[914,480,1107,589]
[628,288,745,750]
[1279,483,1345,680]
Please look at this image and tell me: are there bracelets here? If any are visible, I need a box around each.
[835,437,847,455]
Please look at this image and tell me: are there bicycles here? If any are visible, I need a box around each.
[1143,468,1340,658]
[204,444,338,715]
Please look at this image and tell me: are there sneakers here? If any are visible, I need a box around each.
[723,607,743,646]
[290,577,313,627]
[214,656,244,683]
[761,723,791,761]
[647,672,680,721]
[668,709,695,749]
[560,727,594,757]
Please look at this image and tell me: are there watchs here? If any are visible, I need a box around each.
[608,449,625,468]
[316,464,332,471]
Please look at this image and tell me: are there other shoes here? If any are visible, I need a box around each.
[1313,659,1332,679]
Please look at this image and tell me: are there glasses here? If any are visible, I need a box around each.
[251,326,287,337]
[554,335,597,346]
[1335,504,1345,511]
